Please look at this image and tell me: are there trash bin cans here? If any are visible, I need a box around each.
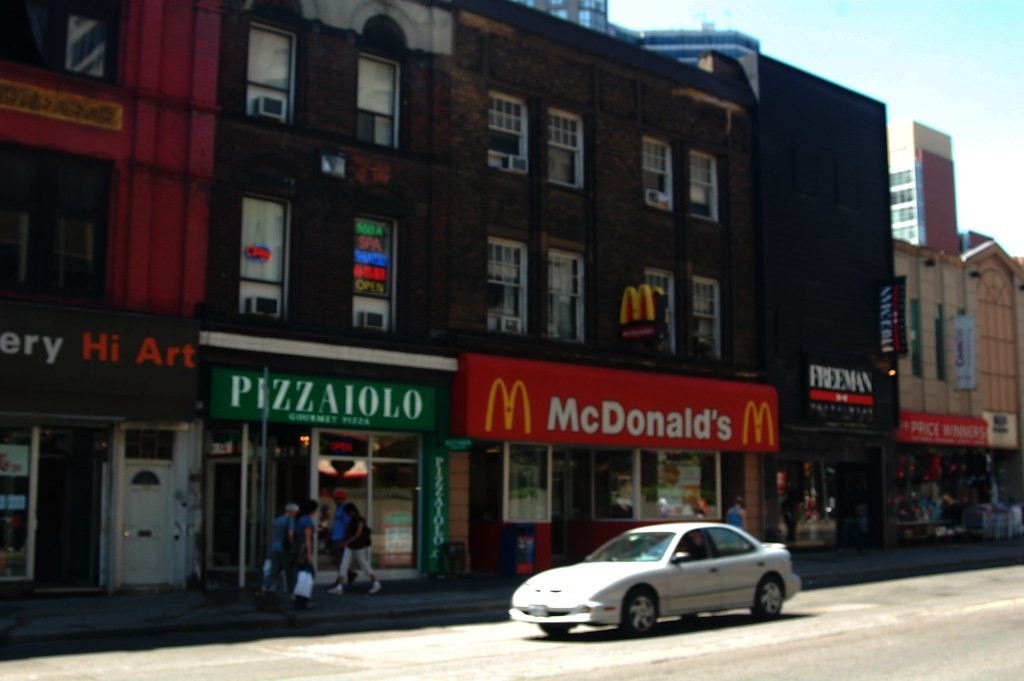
[499,523,536,576]
[433,541,467,580]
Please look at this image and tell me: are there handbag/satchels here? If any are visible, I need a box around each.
[293,570,314,598]
[290,540,308,565]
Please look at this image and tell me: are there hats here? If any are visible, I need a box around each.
[331,491,346,501]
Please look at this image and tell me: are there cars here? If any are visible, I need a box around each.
[507,522,802,638]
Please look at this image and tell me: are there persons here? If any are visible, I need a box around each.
[781,499,798,543]
[675,535,698,562]
[726,491,743,531]
[256,503,299,597]
[289,498,319,606]
[316,504,332,543]
[329,502,382,595]
[938,486,963,527]
[328,489,358,589]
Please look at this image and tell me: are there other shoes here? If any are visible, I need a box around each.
[370,582,381,593]
[329,585,344,594]
